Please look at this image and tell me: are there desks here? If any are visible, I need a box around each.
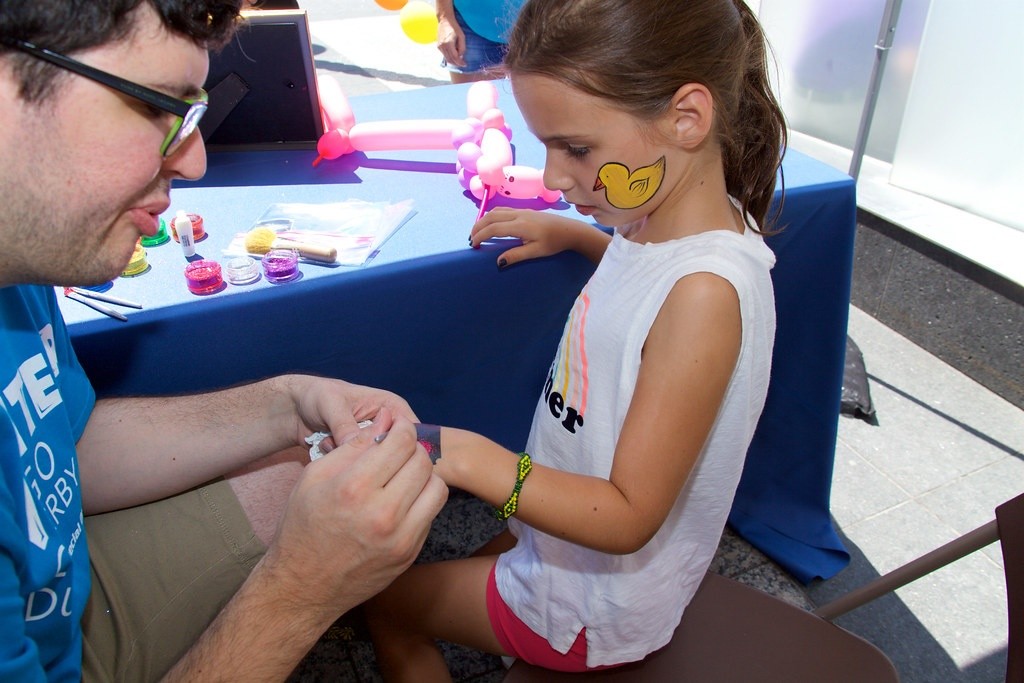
[61,56,862,510]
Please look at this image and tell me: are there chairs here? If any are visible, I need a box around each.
[496,488,1024,683]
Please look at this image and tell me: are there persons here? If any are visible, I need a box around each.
[435,0,527,83]
[0,0,447,683]
[323,0,788,683]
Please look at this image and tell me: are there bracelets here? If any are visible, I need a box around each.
[493,452,532,521]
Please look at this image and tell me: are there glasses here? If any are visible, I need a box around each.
[2,37,211,158]
